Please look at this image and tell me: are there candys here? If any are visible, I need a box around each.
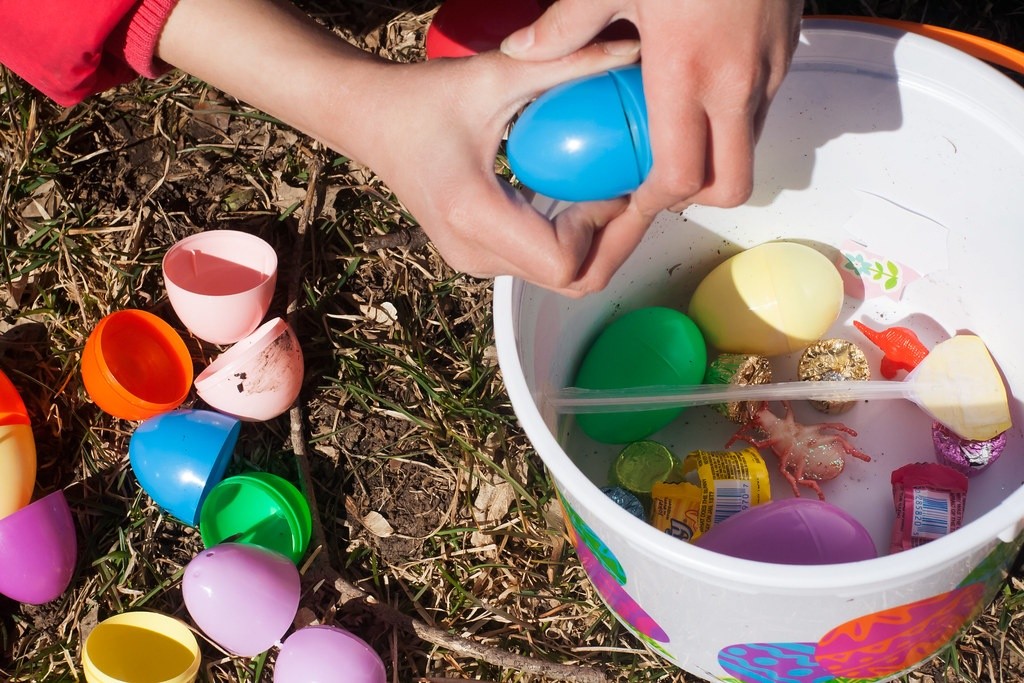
[599,340,1007,552]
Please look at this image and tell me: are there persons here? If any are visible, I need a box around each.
[1,0,804,299]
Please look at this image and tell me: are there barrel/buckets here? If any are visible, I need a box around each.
[493,14,1024,683]
